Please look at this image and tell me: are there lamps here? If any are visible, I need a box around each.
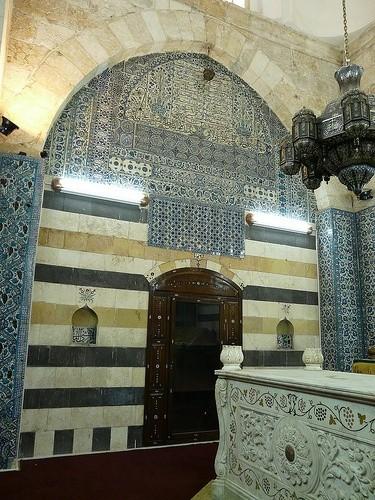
[51,177,151,206]
[246,212,313,235]
[279,0,375,201]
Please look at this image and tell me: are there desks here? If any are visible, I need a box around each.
[214,369,375,500]
